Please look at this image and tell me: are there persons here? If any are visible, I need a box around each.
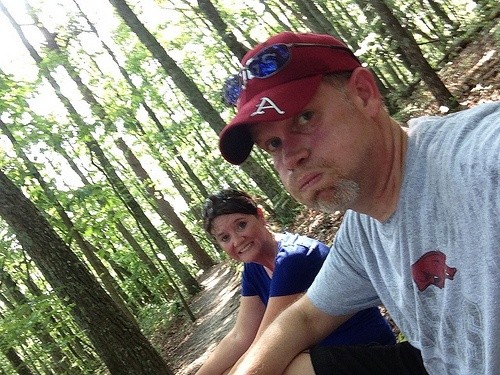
[194,189,397,375]
[218,32,500,375]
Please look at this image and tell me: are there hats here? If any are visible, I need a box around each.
[217,31,362,165]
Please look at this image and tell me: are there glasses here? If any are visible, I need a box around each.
[223,41,360,105]
